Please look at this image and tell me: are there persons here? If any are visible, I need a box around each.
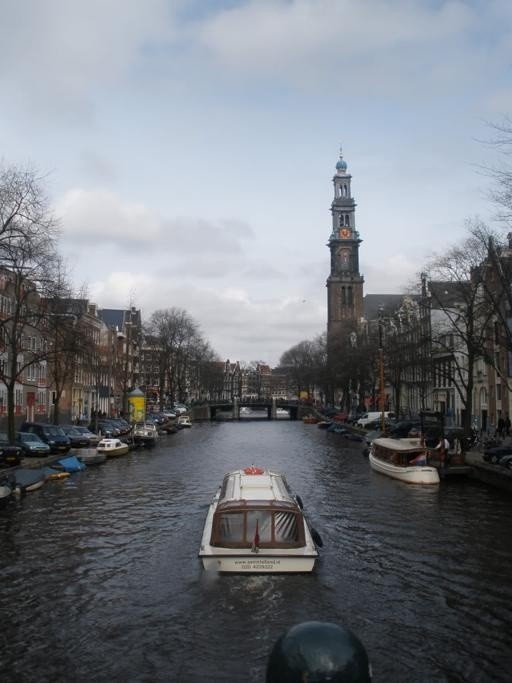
[504,414,511,437]
[497,416,505,440]
[433,434,450,452]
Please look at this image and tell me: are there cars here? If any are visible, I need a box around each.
[146,401,189,424]
[483,446,512,470]
[0,422,100,465]
[303,416,363,442]
[334,412,476,446]
[88,418,132,437]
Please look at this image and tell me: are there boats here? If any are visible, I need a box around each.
[127,421,158,445]
[0,446,107,504]
[96,439,129,456]
[198,461,317,576]
[369,437,440,485]
[158,416,192,435]
